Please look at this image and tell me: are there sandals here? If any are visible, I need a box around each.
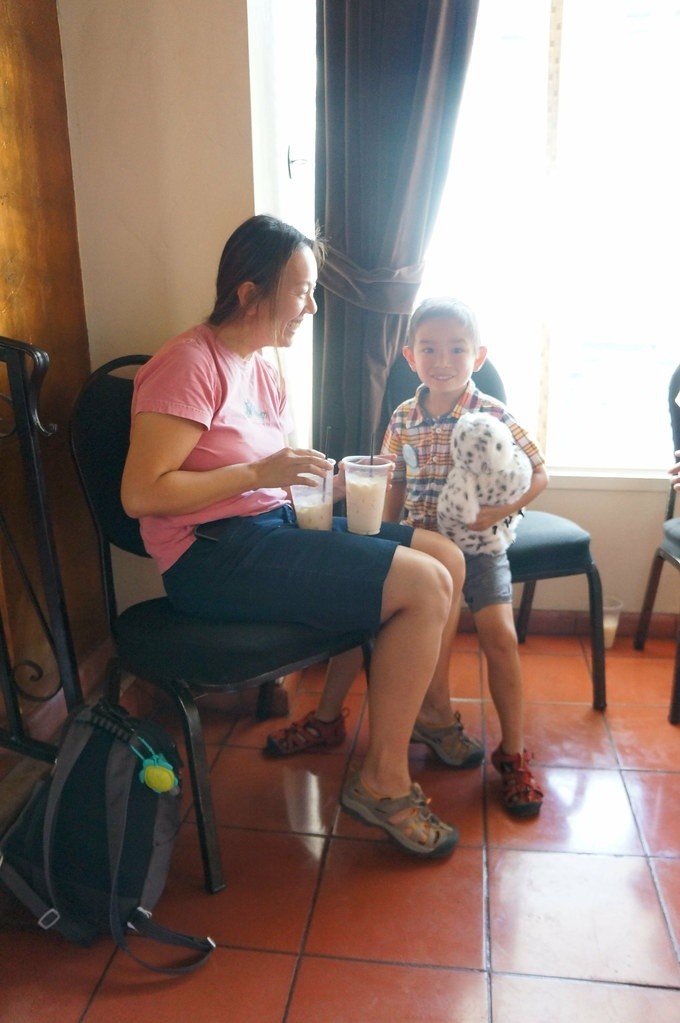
[338,767,458,859]
[267,711,347,757]
[490,743,544,818]
[407,709,485,770]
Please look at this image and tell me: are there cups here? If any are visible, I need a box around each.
[289,456,335,531]
[342,455,392,535]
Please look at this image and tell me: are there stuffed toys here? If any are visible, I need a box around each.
[432,410,536,560]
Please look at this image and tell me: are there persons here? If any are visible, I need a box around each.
[262,295,550,819]
[117,210,490,862]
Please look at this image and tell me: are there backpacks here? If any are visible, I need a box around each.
[0,695,217,977]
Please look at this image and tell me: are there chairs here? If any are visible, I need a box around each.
[67,353,376,894]
[630,363,680,729]
[374,344,611,712]
